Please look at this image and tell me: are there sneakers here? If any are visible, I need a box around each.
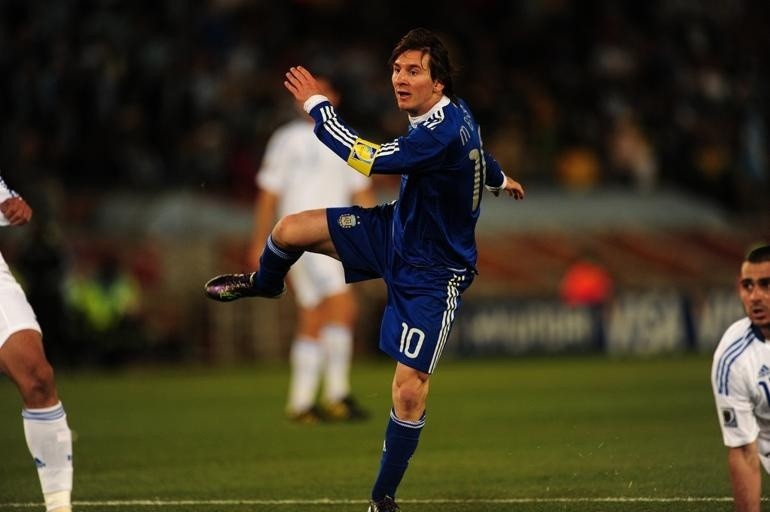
[368,500,400,512]
[203,272,287,301]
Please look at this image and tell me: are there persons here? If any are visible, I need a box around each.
[0,172,73,512]
[710,245,770,512]
[203,27,525,512]
